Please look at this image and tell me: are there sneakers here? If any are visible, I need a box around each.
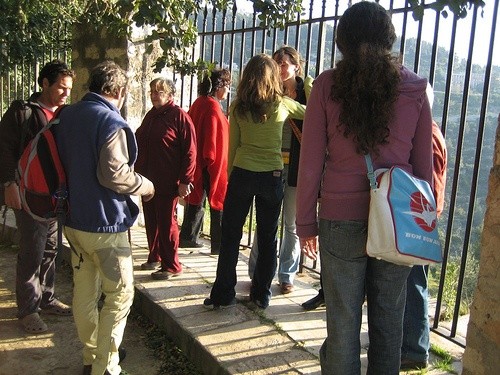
[151,262,182,280]
[141,260,162,270]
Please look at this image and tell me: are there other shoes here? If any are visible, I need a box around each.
[279,282,294,294]
[40,298,72,315]
[180,241,205,248]
[20,313,48,333]
[254,293,270,307]
[204,297,237,310]
[400,359,428,368]
[83,347,129,375]
[211,250,220,254]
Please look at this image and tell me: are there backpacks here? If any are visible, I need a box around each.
[15,104,68,221]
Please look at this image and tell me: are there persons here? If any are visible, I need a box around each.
[177,68,231,255]
[249,46,315,294]
[400,83,447,369]
[134,78,196,279]
[0,59,76,334]
[204,55,315,311]
[58,60,155,375]
[302,270,366,311]
[297,2,433,375]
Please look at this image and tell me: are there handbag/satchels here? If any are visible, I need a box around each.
[366,164,443,267]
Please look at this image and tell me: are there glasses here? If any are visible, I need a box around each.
[147,91,167,96]
